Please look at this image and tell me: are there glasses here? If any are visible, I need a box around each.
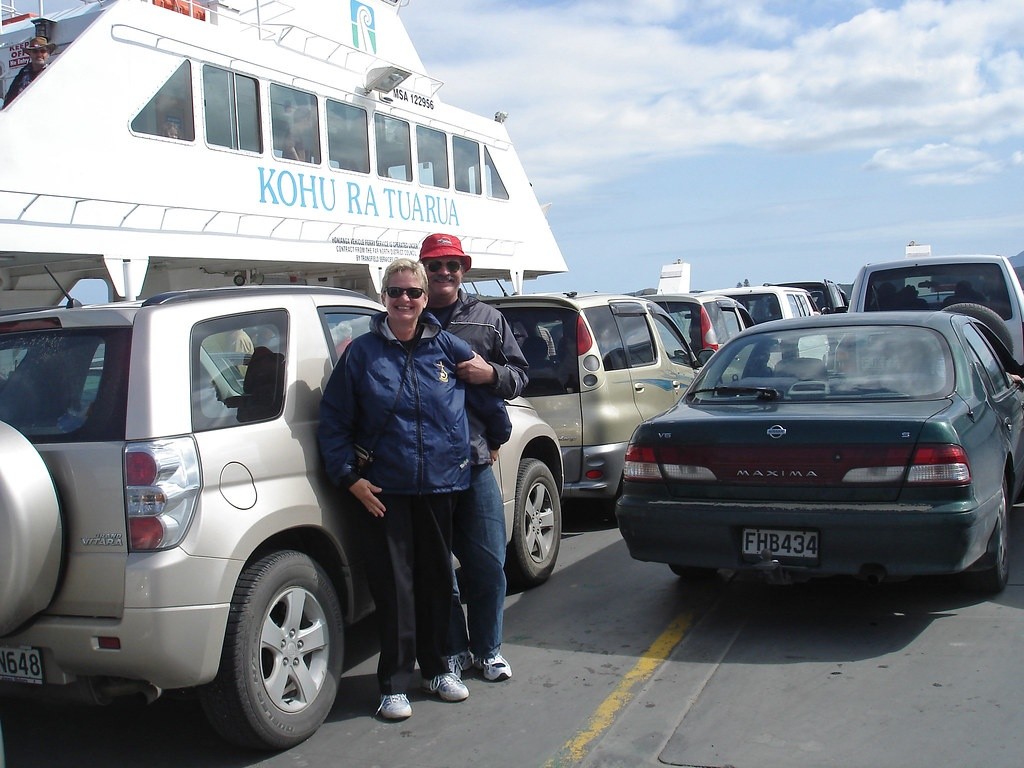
[384,287,426,299]
[427,260,464,273]
[32,48,47,54]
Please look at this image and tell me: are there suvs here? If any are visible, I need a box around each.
[0,284,566,768]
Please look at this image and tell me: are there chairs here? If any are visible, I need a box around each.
[871,284,901,311]
[521,337,554,380]
[951,281,989,308]
[221,345,285,422]
[773,358,830,382]
[808,292,826,312]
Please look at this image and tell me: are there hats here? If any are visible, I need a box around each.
[419,233,472,273]
[24,37,56,56]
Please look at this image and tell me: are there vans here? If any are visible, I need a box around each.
[845,254,1024,373]
[692,277,851,377]
[466,289,717,522]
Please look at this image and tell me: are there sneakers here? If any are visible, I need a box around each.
[474,654,512,682]
[375,692,412,718]
[420,673,469,701]
[449,648,474,677]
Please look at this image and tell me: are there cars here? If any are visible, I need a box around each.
[613,310,1023,599]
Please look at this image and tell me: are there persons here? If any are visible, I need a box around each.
[161,123,179,139]
[282,109,312,162]
[905,285,926,302]
[227,329,254,375]
[2,37,55,108]
[335,325,352,358]
[954,281,971,294]
[318,260,512,718]
[421,233,530,682]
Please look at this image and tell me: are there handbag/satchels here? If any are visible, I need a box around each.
[354,444,374,479]
